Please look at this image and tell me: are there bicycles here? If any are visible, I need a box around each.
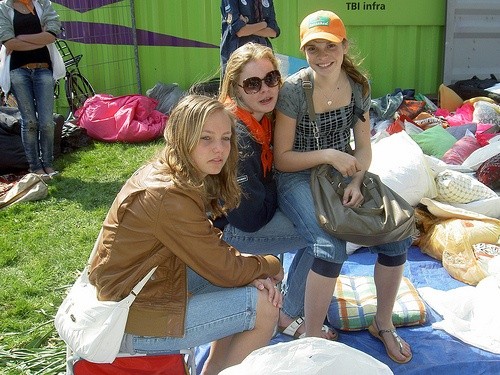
[0,27,98,118]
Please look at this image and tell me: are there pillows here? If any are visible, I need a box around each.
[324,273,427,331]
[398,83,500,203]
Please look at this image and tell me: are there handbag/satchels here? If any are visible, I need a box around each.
[311,164,416,245]
[53,267,136,364]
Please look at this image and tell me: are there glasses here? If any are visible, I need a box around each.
[237,69,281,94]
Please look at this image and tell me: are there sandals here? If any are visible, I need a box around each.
[30,166,59,180]
[279,316,339,341]
[368,316,413,364]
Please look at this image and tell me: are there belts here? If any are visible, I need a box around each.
[20,63,49,69]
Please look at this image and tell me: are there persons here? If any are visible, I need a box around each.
[219,0,280,92]
[0,0,62,182]
[274,9,413,364]
[88,94,284,375]
[214,42,337,343]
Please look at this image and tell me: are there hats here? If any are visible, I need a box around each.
[299,10,346,50]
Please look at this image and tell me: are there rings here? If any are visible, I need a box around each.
[342,173,348,177]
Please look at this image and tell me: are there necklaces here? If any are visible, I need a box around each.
[318,76,344,106]
